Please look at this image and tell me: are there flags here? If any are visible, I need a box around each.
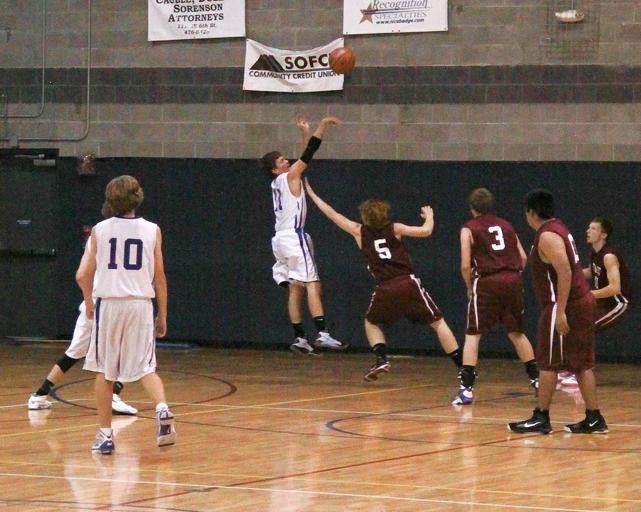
[341,1,455,37]
[145,1,250,44]
[241,36,349,97]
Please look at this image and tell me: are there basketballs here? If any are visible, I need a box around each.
[329,48,354,74]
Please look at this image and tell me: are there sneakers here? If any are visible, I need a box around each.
[533,370,581,395]
[563,413,609,435]
[507,417,552,435]
[453,369,479,404]
[156,407,175,447]
[91,429,118,454]
[364,361,393,382]
[288,332,347,357]
[112,394,138,416]
[29,396,51,410]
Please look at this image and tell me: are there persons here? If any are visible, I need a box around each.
[81,176,179,452]
[451,186,551,406]
[263,112,352,357]
[556,215,634,391]
[301,172,480,381]
[27,197,139,415]
[506,187,610,437]
